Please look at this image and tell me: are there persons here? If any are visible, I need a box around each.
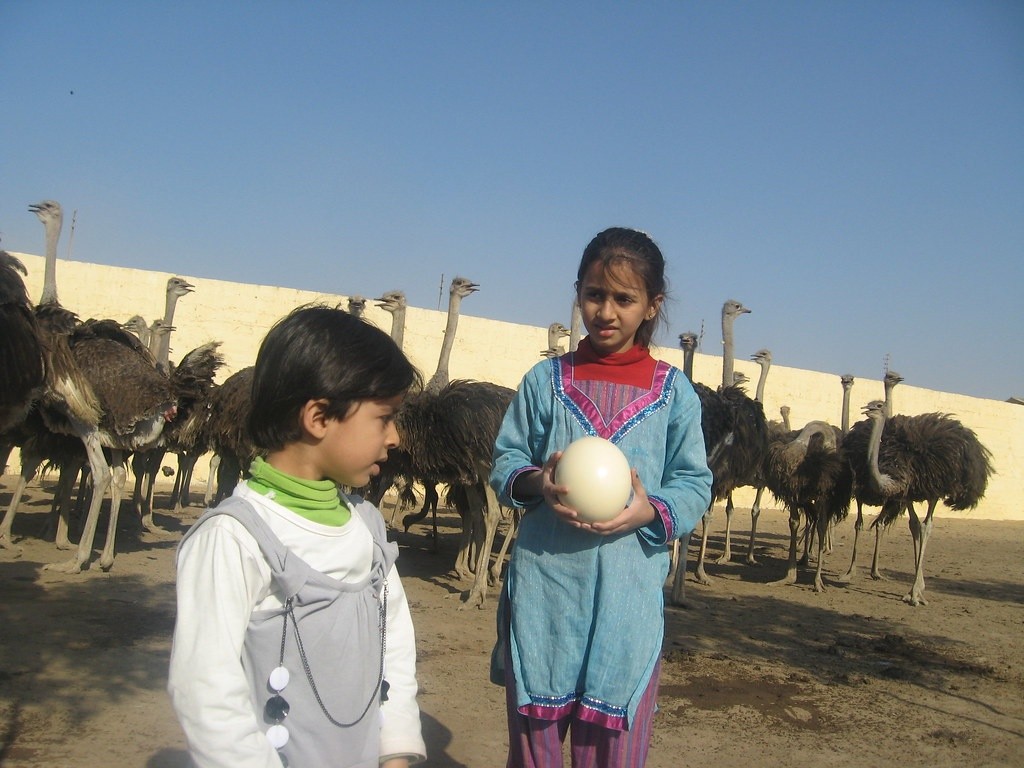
[488,226,715,767]
[163,305,429,768]
